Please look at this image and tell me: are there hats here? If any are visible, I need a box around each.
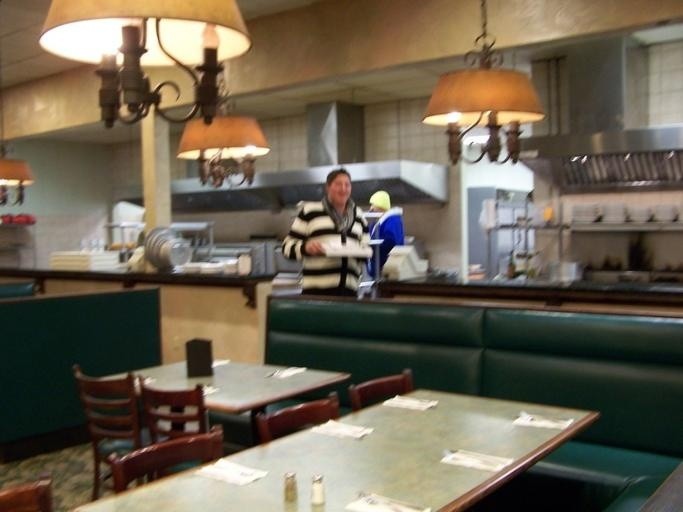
[369,190,391,212]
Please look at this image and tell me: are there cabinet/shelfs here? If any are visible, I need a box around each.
[0,215,39,270]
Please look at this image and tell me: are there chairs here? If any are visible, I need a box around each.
[0,473,53,511]
[108,429,226,493]
[73,363,169,501]
[348,367,414,411]
[138,375,240,482]
[0,280,37,299]
[257,390,340,443]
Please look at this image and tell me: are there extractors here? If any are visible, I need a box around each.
[508,35,681,195]
[169,99,450,214]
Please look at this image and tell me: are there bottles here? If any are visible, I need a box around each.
[309,473,325,507]
[283,471,297,503]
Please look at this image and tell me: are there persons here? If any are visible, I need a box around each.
[368,190,403,278]
[281,168,375,296]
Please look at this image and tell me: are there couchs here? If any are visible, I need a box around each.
[207,294,683,511]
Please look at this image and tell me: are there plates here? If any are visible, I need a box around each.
[143,224,193,270]
[570,201,679,225]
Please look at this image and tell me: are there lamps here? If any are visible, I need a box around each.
[422,1,547,168]
[0,141,35,206]
[38,0,252,127]
[176,63,270,187]
